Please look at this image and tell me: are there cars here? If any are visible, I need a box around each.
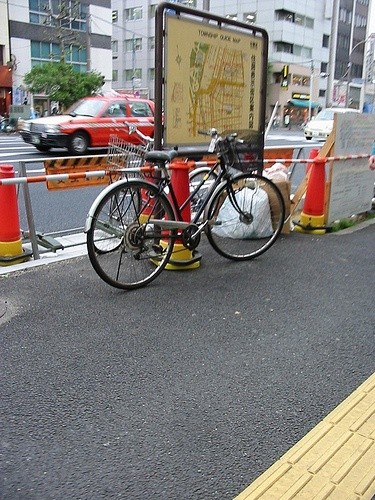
[303,108,363,141]
[19,91,164,155]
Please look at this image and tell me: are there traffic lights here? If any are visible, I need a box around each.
[279,64,290,90]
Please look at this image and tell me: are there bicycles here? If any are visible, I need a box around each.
[84,128,285,289]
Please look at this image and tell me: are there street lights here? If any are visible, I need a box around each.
[345,1,375,109]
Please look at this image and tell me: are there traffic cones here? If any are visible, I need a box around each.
[294,149,326,234]
[150,156,200,270]
[138,160,163,224]
[0,164,33,265]
